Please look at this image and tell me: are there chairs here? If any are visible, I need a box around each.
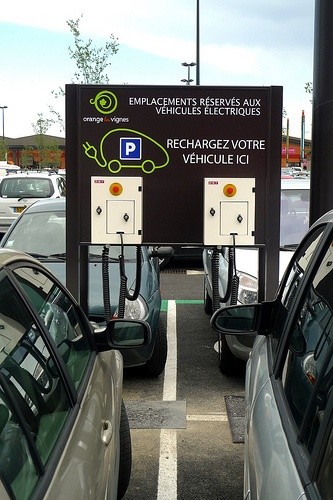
[292,201,308,221]
[45,216,65,226]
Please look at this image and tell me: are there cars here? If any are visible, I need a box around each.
[1,248,132,498]
[282,167,310,179]
[0,199,161,376]
[42,168,55,174]
[1,164,21,175]
[58,168,65,175]
[202,179,311,378]
[292,165,301,175]
[210,209,333,499]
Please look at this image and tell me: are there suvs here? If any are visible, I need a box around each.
[0,170,65,236]
